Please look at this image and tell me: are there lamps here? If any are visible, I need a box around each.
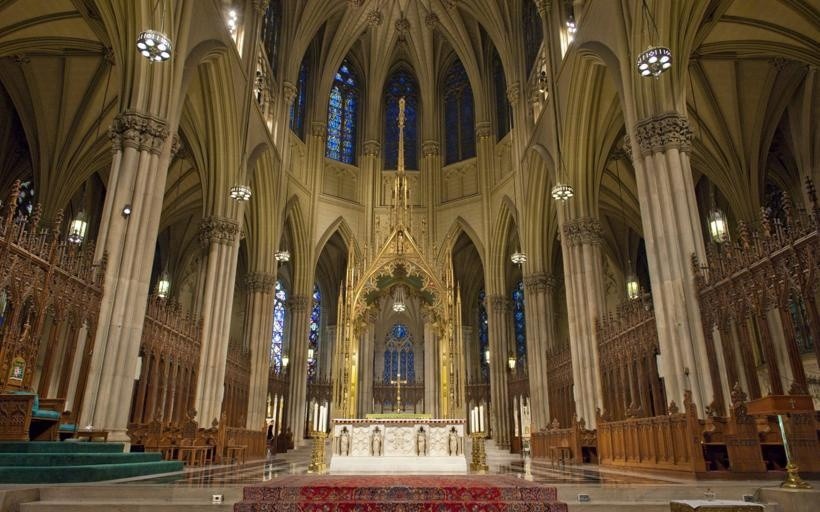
[636,0,672,78]
[68,59,113,246]
[230,14,259,202]
[510,143,527,264]
[688,64,731,246]
[507,349,516,370]
[275,102,289,263]
[157,157,183,299]
[392,285,408,312]
[543,0,575,201]
[136,0,173,63]
[121,204,132,219]
[615,157,640,301]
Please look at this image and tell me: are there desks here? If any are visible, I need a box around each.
[550,446,572,466]
[76,431,248,468]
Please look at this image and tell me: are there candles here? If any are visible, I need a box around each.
[777,415,794,469]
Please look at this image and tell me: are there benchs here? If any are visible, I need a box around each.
[31,392,78,440]
